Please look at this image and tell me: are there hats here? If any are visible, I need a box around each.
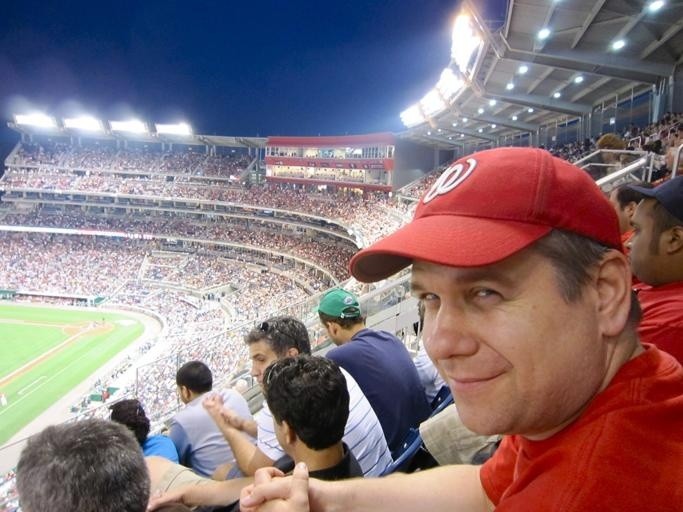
[349,147,622,284]
[311,288,362,318]
[626,175,683,219]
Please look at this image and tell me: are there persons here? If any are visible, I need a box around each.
[0,111,682,511]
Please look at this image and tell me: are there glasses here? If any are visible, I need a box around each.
[258,321,298,346]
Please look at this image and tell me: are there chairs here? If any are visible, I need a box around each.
[231,382,452,512]
